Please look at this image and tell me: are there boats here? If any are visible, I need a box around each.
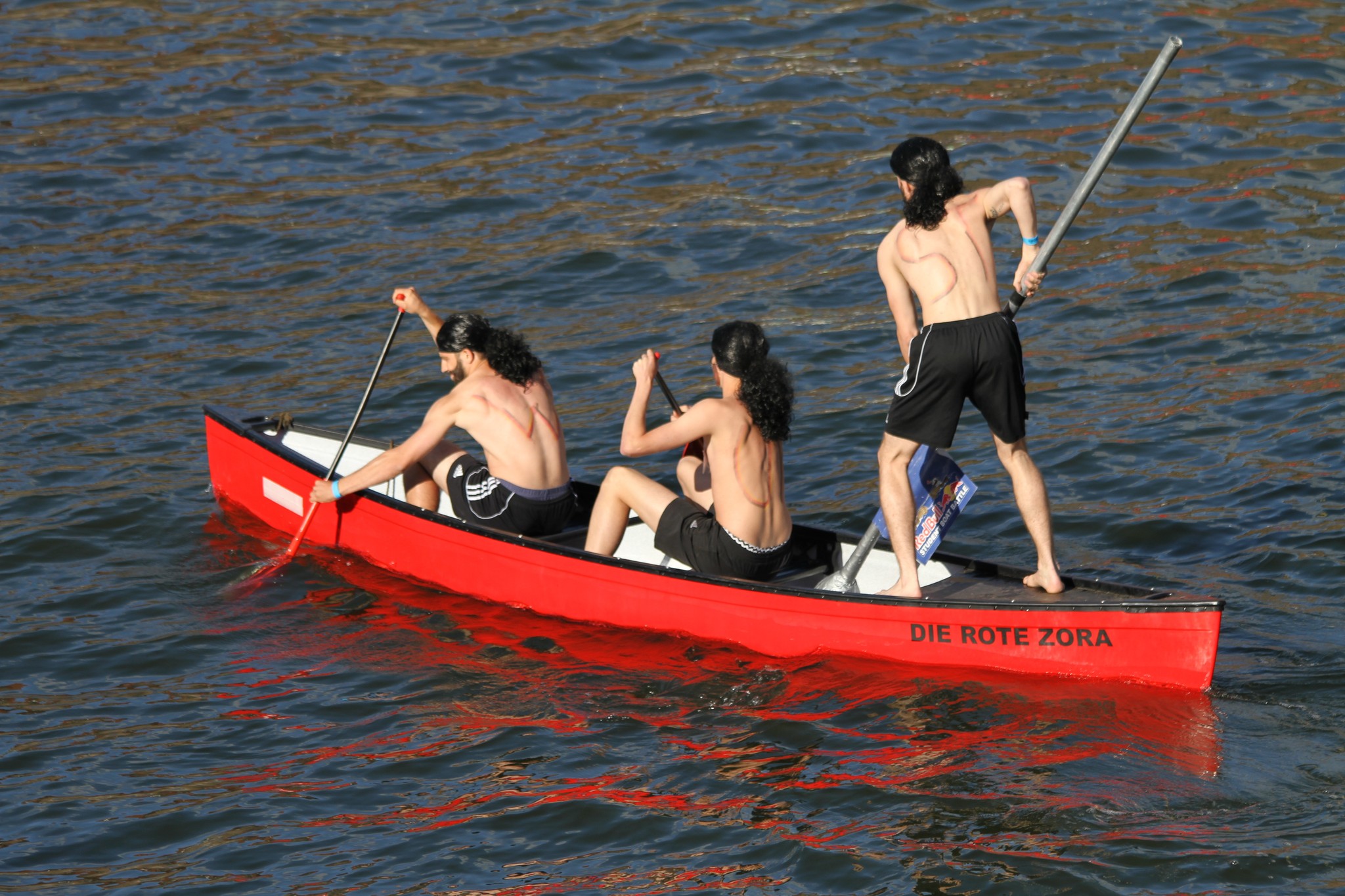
[203,402,1226,702]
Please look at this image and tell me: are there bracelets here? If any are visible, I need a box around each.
[1022,235,1039,246]
[331,480,343,499]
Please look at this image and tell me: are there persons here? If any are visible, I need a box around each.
[308,286,578,538]
[875,136,1065,600]
[583,320,795,583]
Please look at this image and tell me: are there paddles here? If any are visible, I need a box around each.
[227,290,407,600]
[640,351,704,464]
